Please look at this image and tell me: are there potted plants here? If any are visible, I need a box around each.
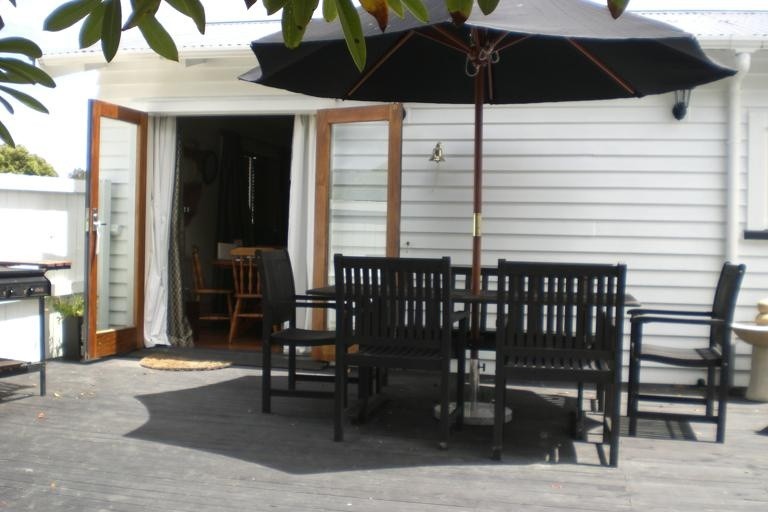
[48,292,87,362]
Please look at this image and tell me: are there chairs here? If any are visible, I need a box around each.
[186,247,278,351]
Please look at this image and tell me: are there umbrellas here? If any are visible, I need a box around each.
[237,1,738,404]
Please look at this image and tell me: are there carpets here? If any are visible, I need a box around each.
[138,352,233,371]
